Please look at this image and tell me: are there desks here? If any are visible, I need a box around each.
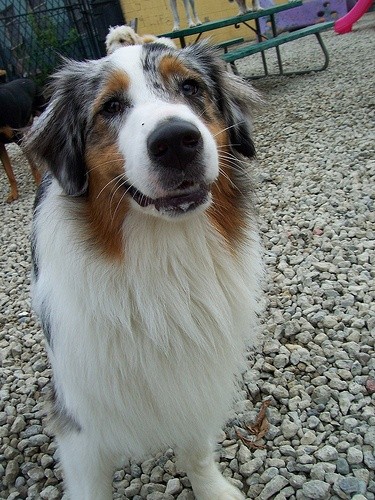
[156,0,303,76]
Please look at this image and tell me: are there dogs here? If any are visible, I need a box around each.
[0,69,49,204]
[11,24,274,500]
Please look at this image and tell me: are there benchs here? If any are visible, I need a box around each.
[220,21,336,77]
[214,37,244,76]
